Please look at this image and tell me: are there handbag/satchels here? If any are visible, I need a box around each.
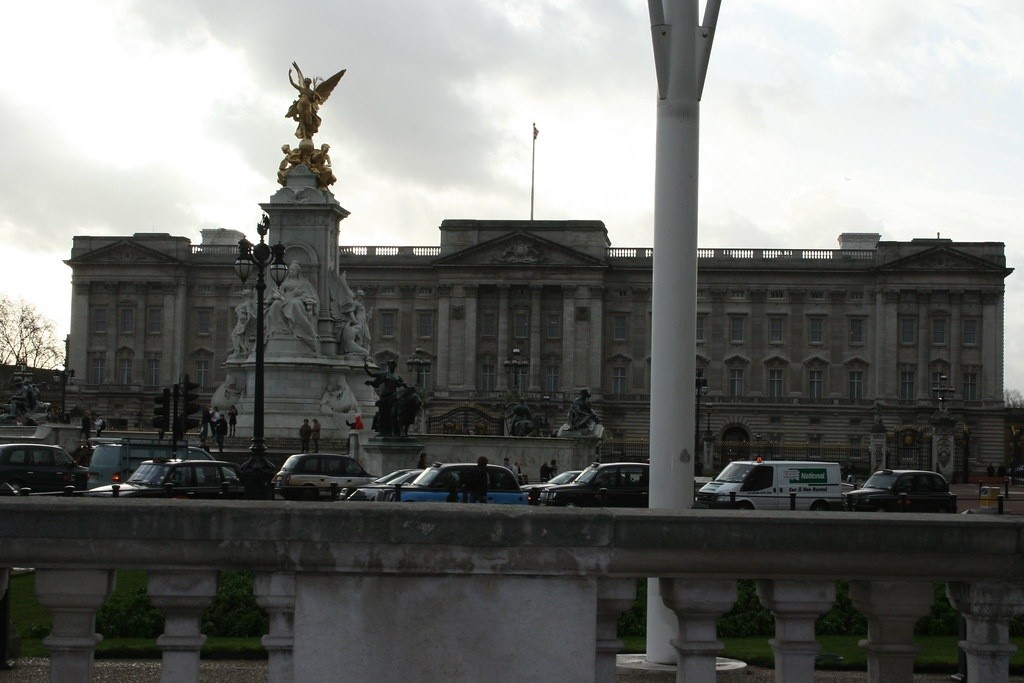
[98,418,106,430]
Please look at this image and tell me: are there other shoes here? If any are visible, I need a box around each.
[219,449,223,452]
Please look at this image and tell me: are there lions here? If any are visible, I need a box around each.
[374,386,424,439]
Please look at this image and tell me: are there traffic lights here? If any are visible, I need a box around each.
[154,387,170,432]
[184,381,200,432]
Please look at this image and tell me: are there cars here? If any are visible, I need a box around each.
[91,458,244,500]
[271,453,380,501]
[0,443,90,497]
[0,481,18,495]
[520,470,582,505]
[374,461,529,505]
[841,467,957,513]
[540,460,649,507]
[340,468,423,501]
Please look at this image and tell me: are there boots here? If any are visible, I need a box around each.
[232,429,235,438]
[228,429,232,437]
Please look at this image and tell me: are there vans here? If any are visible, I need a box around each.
[87,438,238,490]
[698,459,841,510]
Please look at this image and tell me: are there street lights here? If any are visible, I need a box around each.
[233,217,290,500]
[406,345,431,393]
[695,369,708,476]
[504,344,529,386]
[53,368,75,422]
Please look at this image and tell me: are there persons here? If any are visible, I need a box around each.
[266,262,319,352]
[416,452,426,469]
[349,289,371,349]
[17,404,238,452]
[504,457,558,486]
[568,389,600,430]
[6,379,29,418]
[278,145,302,186]
[504,397,534,435]
[231,290,250,357]
[364,359,408,427]
[307,144,331,192]
[468,457,489,503]
[345,417,364,454]
[289,70,321,139]
[299,419,321,453]
